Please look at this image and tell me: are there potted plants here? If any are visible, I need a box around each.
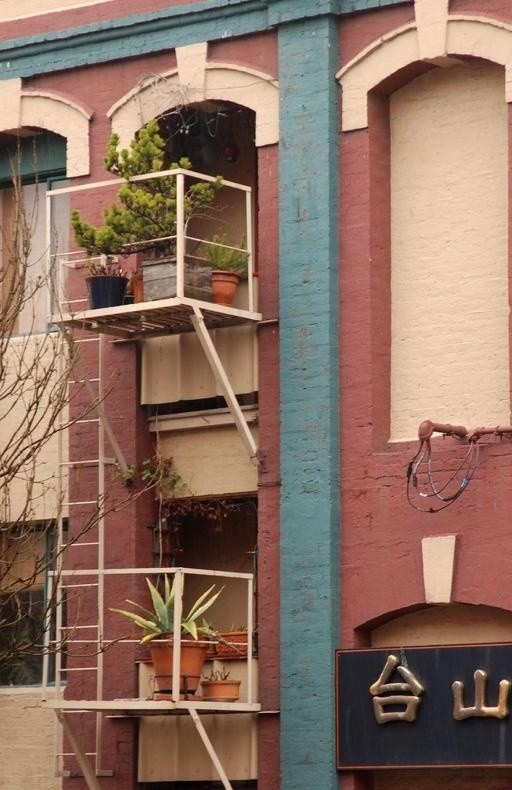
[196,233,250,307]
[201,671,241,702]
[84,263,129,309]
[216,623,248,656]
[70,119,215,302]
[108,573,226,694]
